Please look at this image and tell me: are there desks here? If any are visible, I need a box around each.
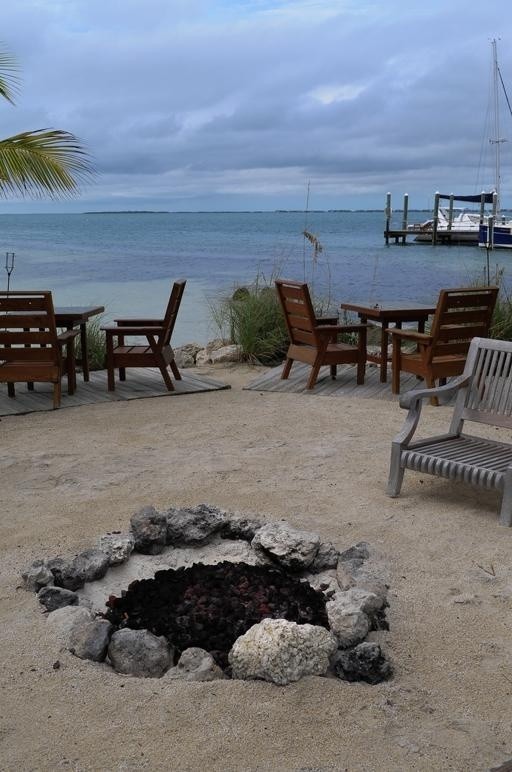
[18,305,104,391]
[340,300,437,384]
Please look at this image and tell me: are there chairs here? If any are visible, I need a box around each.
[99,278,187,392]
[384,286,512,527]
[274,279,373,391]
[0,290,83,411]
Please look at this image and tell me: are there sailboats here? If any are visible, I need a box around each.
[414,33,512,248]
